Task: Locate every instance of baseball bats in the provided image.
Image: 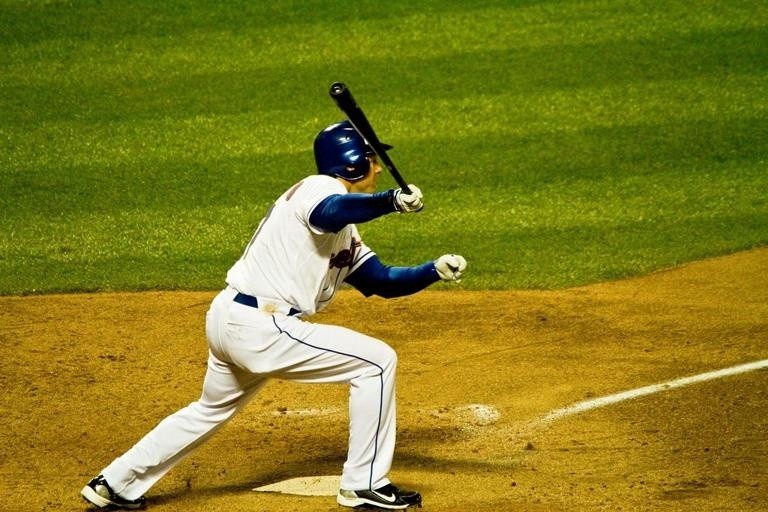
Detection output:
[329,81,412,195]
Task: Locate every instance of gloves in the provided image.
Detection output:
[393,183,425,214]
[434,253,468,285]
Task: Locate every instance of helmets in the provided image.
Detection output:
[313,120,394,183]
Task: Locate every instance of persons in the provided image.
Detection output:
[80,120,470,511]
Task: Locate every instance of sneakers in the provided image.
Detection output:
[336,482,423,511]
[80,471,146,509]
